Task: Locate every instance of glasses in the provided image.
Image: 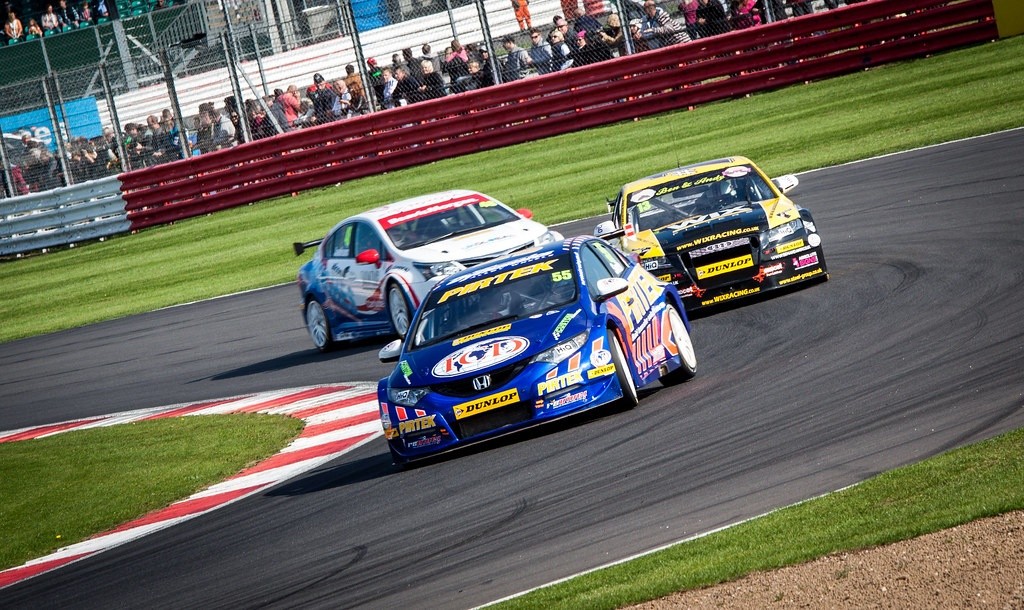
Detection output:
[553,36,557,38]
[556,24,566,28]
[531,34,540,38]
[576,38,581,41]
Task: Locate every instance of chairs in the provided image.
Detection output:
[8,0,172,45]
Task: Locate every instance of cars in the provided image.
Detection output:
[292,189,565,352]
[593,155,828,316]
[377,235,697,466]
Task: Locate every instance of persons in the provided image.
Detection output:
[190,45,447,177]
[706,181,737,209]
[440,0,626,131]
[0,109,192,199]
[478,291,511,317]
[5,0,110,45]
[629,0,926,89]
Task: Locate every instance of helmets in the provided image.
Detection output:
[478,285,521,323]
[386,224,408,247]
[706,181,738,211]
[629,19,643,28]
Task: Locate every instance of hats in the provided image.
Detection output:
[314,73,324,84]
[368,57,377,66]
[479,45,488,53]
[576,30,587,38]
